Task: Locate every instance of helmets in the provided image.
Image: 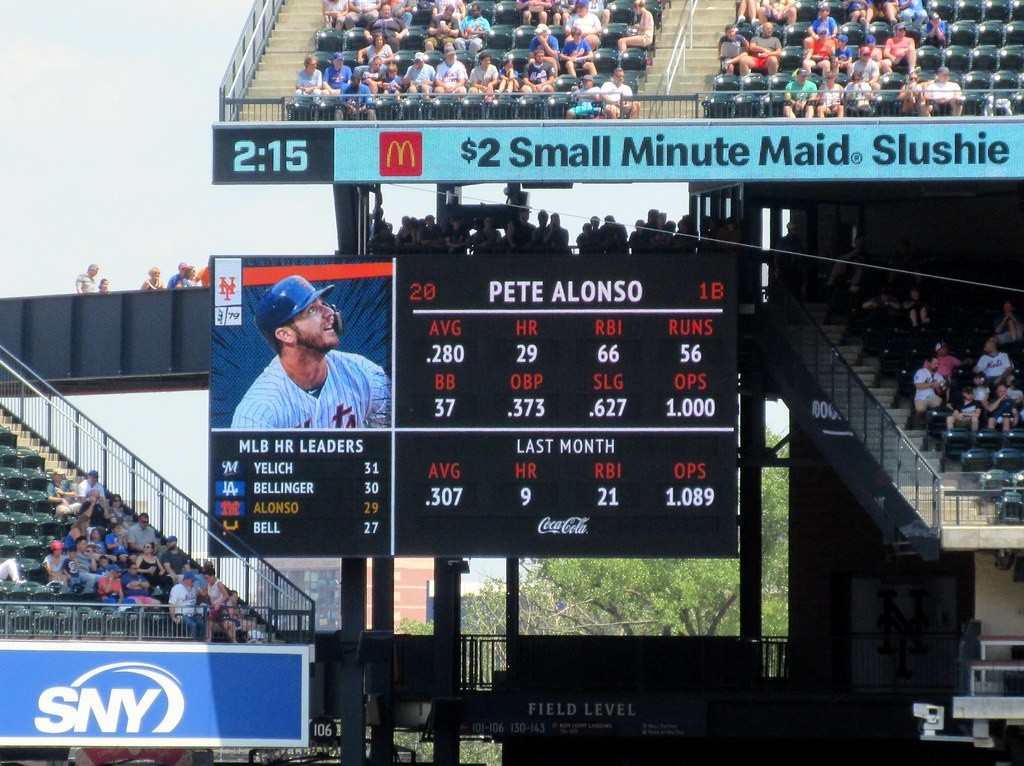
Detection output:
[251,273,335,334]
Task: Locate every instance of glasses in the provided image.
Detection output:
[864,53,870,56]
[910,78,918,82]
[899,28,906,31]
[143,545,150,549]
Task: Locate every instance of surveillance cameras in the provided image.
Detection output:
[913,704,939,718]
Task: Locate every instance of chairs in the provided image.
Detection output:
[285,0,665,129]
[813,301,1024,527]
[0,420,174,631]
[702,0,1024,117]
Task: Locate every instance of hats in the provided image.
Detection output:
[575,0,588,7]
[165,535,177,542]
[50,541,63,550]
[444,46,457,56]
[333,52,345,61]
[817,4,831,10]
[895,24,906,31]
[414,51,425,62]
[178,261,188,270]
[183,571,200,581]
[534,24,549,33]
[796,68,807,76]
[837,35,848,42]
[817,25,827,34]
[865,34,876,46]
[108,563,123,572]
[860,47,870,54]
[928,10,940,20]
[937,67,950,75]
[87,470,100,478]
[578,74,594,82]
[52,467,66,475]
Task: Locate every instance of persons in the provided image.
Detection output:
[75,264,99,293]
[0,558,27,584]
[567,75,607,119]
[516,0,611,34]
[600,67,641,119]
[167,263,198,288]
[783,0,965,117]
[287,46,558,106]
[43,468,262,644]
[576,210,744,254]
[718,21,782,76]
[322,0,418,53]
[736,0,797,33]
[368,206,572,255]
[564,0,602,52]
[194,266,210,286]
[98,278,110,293]
[559,27,597,75]
[618,0,654,52]
[528,24,560,74]
[228,274,391,429]
[141,267,165,289]
[334,73,376,120]
[772,224,1024,433]
[422,0,491,51]
